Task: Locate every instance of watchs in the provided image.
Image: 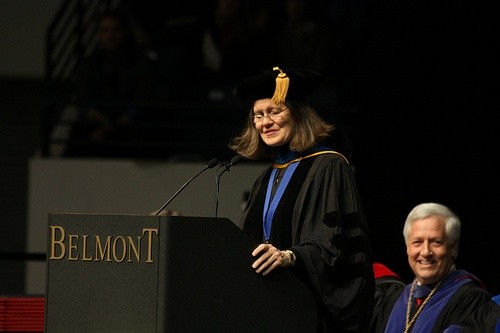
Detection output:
[286,249,297,265]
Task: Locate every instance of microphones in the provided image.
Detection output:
[156,159,217,215]
[215,155,242,218]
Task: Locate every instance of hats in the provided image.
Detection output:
[240,70,321,101]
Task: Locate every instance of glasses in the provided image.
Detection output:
[252,107,289,118]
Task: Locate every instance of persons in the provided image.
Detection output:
[73,0,332,154]
[364,202,500,332]
[228,71,377,333]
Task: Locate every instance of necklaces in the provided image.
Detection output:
[405,278,441,333]
